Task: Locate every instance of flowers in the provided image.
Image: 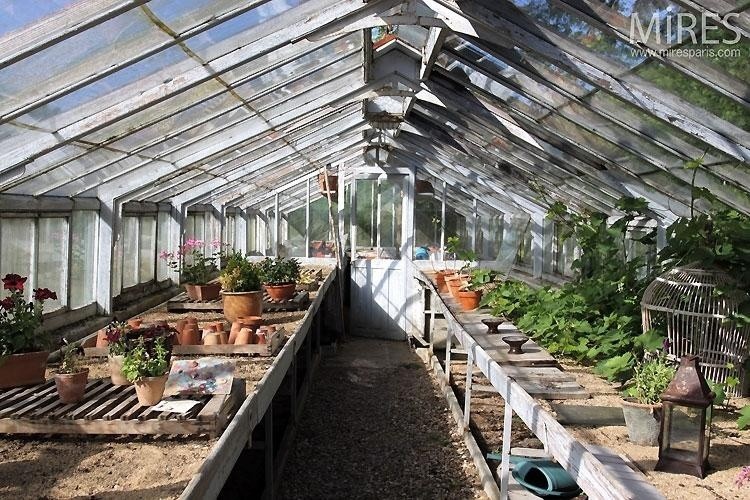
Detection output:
[618,337,681,405]
[159,238,223,285]
[0,273,57,354]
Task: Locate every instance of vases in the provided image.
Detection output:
[0,351,50,388]
[184,282,203,301]
[620,401,663,446]
[194,285,219,300]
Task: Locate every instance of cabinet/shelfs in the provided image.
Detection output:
[0,261,336,499]
[415,274,656,500]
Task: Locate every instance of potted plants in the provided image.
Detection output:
[444,247,482,299]
[52,337,90,403]
[458,266,506,311]
[258,255,304,300]
[103,321,134,385]
[437,231,463,293]
[216,247,263,325]
[118,334,173,407]
